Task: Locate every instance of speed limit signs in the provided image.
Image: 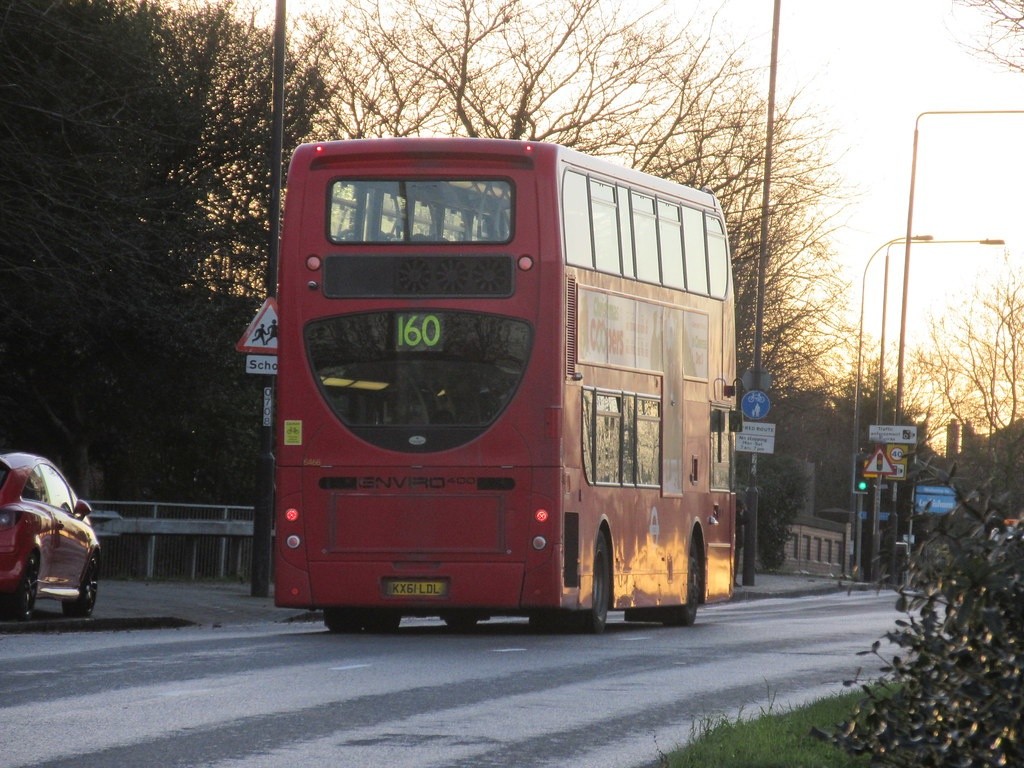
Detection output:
[884,444,908,481]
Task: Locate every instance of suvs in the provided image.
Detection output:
[0,451,103,622]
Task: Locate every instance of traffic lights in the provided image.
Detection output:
[852,453,869,495]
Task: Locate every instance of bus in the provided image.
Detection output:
[265,138,748,636]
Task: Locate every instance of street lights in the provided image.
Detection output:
[843,233,937,582]
[867,236,1007,581]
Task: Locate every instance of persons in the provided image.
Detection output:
[733,499,752,589]
